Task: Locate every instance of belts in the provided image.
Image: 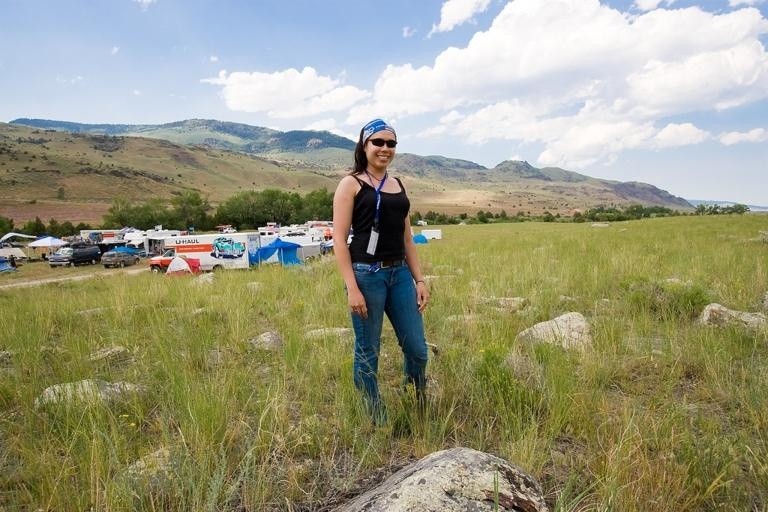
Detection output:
[375,258,406,271]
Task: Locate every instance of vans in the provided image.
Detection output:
[49,245,101,268]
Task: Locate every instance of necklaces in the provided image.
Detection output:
[367,170,388,182]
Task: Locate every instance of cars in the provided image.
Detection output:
[100,250,140,268]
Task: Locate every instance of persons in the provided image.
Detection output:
[332,120,430,437]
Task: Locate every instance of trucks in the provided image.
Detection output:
[149,232,262,280]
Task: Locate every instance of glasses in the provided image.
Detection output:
[367,137,398,148]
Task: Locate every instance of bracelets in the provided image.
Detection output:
[416,280,425,284]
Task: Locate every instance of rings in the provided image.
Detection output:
[355,310,358,312]
